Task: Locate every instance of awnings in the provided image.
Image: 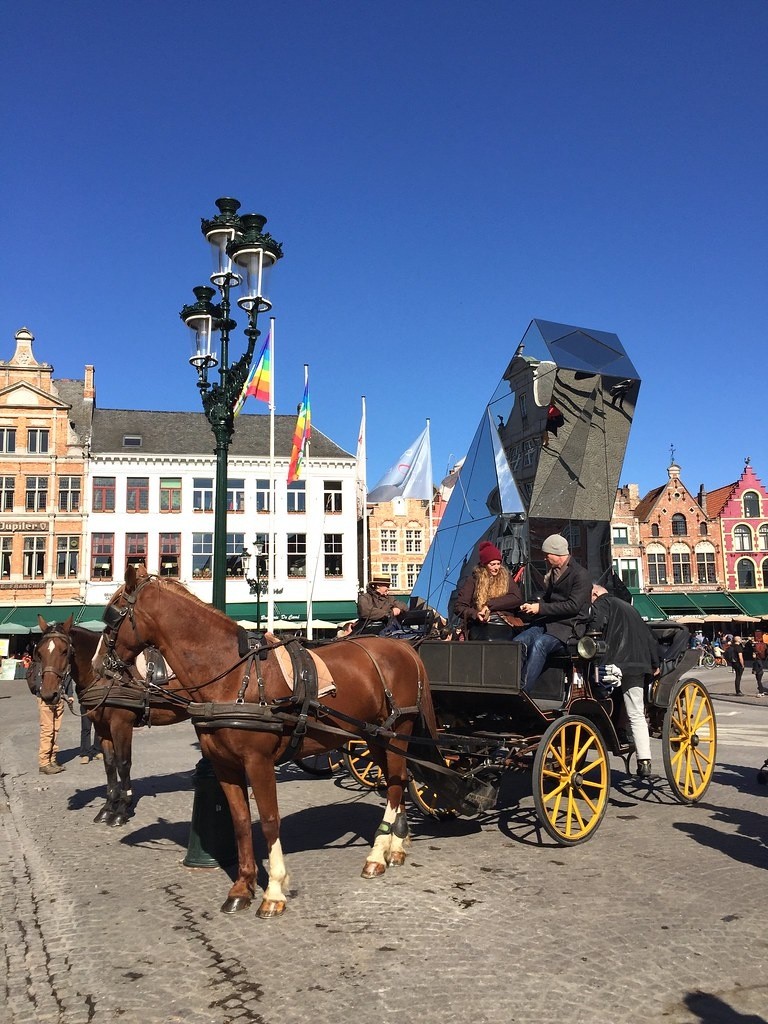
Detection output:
[629,593,768,623]
[0,606,107,634]
[226,603,360,629]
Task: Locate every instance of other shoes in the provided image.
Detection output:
[756,693,764,698]
[39,764,61,775]
[95,753,103,760]
[80,756,90,764]
[51,761,66,771]
[736,691,746,696]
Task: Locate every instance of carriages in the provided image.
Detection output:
[31,564,718,921]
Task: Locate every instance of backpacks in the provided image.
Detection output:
[723,646,735,666]
[26,661,38,694]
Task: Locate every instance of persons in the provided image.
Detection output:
[691,630,765,698]
[513,534,593,696]
[453,541,524,641]
[336,622,353,638]
[79,701,104,764]
[22,653,32,680]
[9,654,15,659]
[35,643,74,775]
[587,584,661,778]
[351,575,409,636]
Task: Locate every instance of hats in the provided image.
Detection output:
[541,533,570,555]
[714,642,719,645]
[478,541,502,566]
[369,574,392,585]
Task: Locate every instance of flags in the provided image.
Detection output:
[286,373,311,486]
[367,425,433,502]
[233,329,272,419]
[355,412,366,503]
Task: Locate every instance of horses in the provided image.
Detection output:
[35,610,256,827]
[91,563,440,919]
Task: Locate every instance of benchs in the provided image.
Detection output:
[645,620,691,676]
[520,637,580,700]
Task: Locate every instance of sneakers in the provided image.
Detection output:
[637,759,652,776]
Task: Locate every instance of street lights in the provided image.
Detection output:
[179,195,285,621]
